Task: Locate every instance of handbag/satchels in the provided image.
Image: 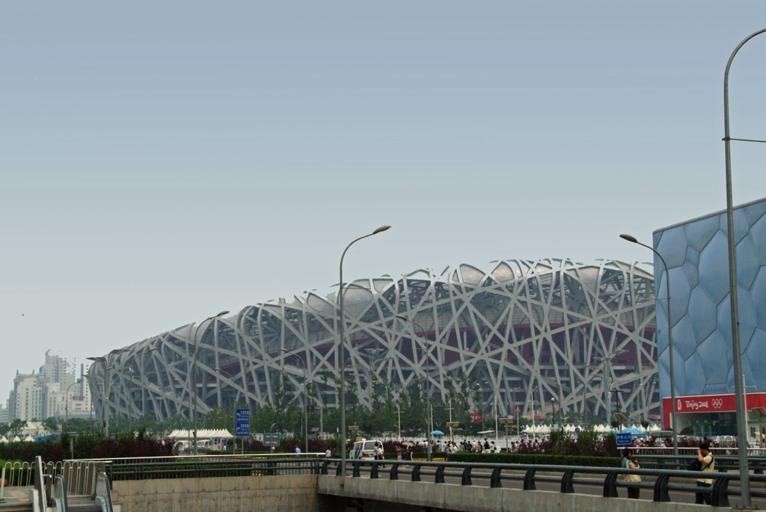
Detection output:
[624,460,642,483]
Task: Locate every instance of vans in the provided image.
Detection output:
[349,439,386,466]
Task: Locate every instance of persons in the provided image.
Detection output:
[374,442,385,468]
[621,448,641,499]
[325,445,332,458]
[628,436,766,454]
[393,437,545,461]
[695,441,714,504]
[295,444,302,467]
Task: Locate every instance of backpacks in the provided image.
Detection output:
[689,454,714,471]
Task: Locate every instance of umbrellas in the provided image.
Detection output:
[431,430,445,439]
[753,432,766,440]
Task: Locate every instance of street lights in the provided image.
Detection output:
[278,346,308,458]
[394,314,432,465]
[618,230,682,470]
[126,346,161,439]
[213,365,245,453]
[63,378,81,421]
[336,223,393,469]
[549,396,556,426]
[190,309,231,456]
